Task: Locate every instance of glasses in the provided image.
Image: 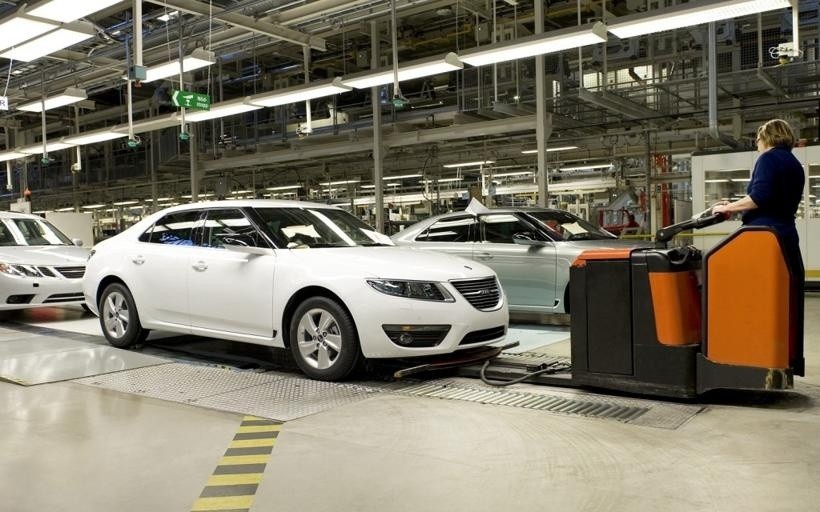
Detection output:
[755,139,760,145]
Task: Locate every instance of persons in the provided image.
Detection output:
[711,119,804,388]
[627,214,641,227]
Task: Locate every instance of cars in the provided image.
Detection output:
[82,199,509,381]
[1,210,94,316]
[391,206,652,315]
